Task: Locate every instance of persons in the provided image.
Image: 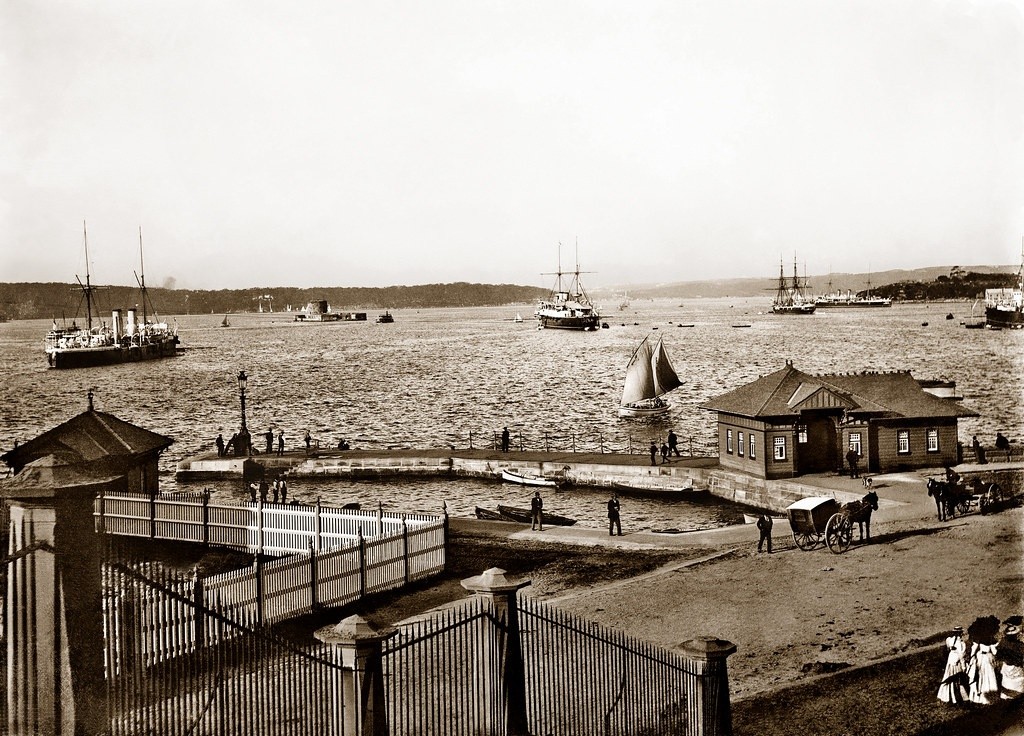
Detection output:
[650,430,680,465]
[757,508,774,554]
[846,450,858,479]
[277,432,285,456]
[266,428,273,453]
[532,492,544,531]
[972,436,980,451]
[936,626,1024,705]
[607,493,624,536]
[304,430,311,448]
[995,432,1009,449]
[249,478,287,504]
[502,427,509,452]
[216,434,224,457]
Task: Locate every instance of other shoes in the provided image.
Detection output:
[539,529,545,531]
[667,455,671,457]
[610,534,616,536]
[768,550,773,554]
[652,463,656,466]
[677,454,680,457]
[758,547,761,549]
[532,528,534,530]
[618,534,625,536]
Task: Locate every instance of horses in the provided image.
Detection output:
[927,477,1005,522]
[837,492,878,545]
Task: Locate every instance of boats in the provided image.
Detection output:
[290,495,361,514]
[501,464,572,488]
[474,505,519,523]
[813,262,894,308]
[497,502,578,527]
[375,310,394,324]
[983,234,1024,330]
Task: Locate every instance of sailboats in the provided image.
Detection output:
[532,233,601,331]
[760,249,818,316]
[42,215,181,371]
[616,330,689,420]
[511,312,525,324]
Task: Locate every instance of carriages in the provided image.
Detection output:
[927,474,1004,522]
[786,491,879,555]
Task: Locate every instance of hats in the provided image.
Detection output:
[503,427,507,429]
[535,492,540,495]
[953,626,963,632]
[1005,626,1020,635]
[651,442,655,444]
[669,430,673,432]
